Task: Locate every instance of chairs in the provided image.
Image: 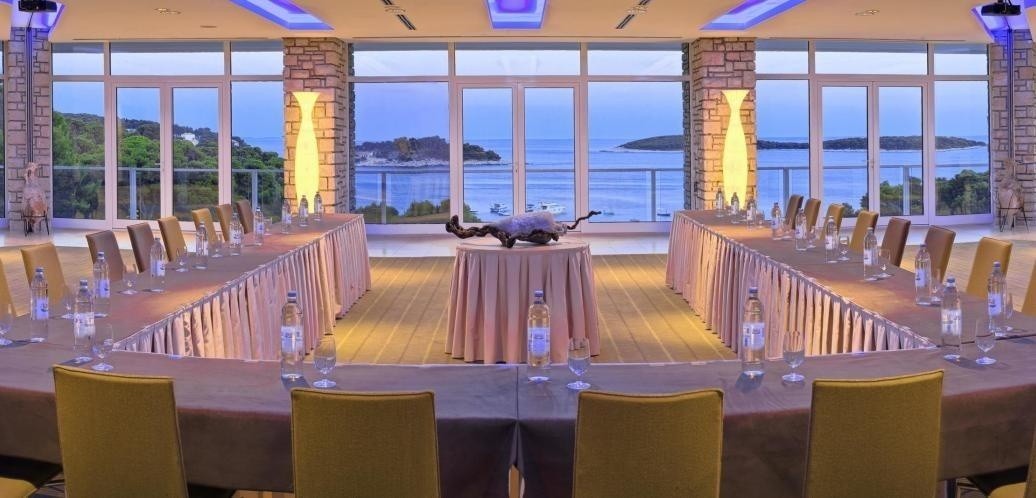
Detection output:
[571,389,724,497]
[51,364,189,498]
[989,428,1036,496]
[804,368,943,498]
[783,194,1036,317]
[0,477,38,498]
[0,199,255,318]
[289,386,440,498]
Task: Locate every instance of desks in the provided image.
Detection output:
[0,213,372,361]
[1,348,519,497]
[665,210,1036,358]
[514,335,1036,497]
[442,241,602,364]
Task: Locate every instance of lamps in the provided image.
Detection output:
[719,88,752,211]
[292,90,322,216]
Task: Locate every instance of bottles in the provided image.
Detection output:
[0,192,337,390]
[771,202,783,241]
[741,287,766,377]
[731,191,740,224]
[796,207,809,250]
[717,190,725,217]
[864,227,878,281]
[528,291,550,383]
[914,244,933,305]
[940,278,963,359]
[987,262,1007,337]
[825,215,839,265]
[748,198,756,230]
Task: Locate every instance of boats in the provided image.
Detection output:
[656,211,670,216]
[491,201,566,216]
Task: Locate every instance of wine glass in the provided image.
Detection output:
[782,332,806,382]
[1006,293,1013,331]
[838,236,850,261]
[806,225,816,248]
[878,248,892,280]
[931,269,942,303]
[726,203,791,233]
[566,337,591,390]
[973,318,996,366]
